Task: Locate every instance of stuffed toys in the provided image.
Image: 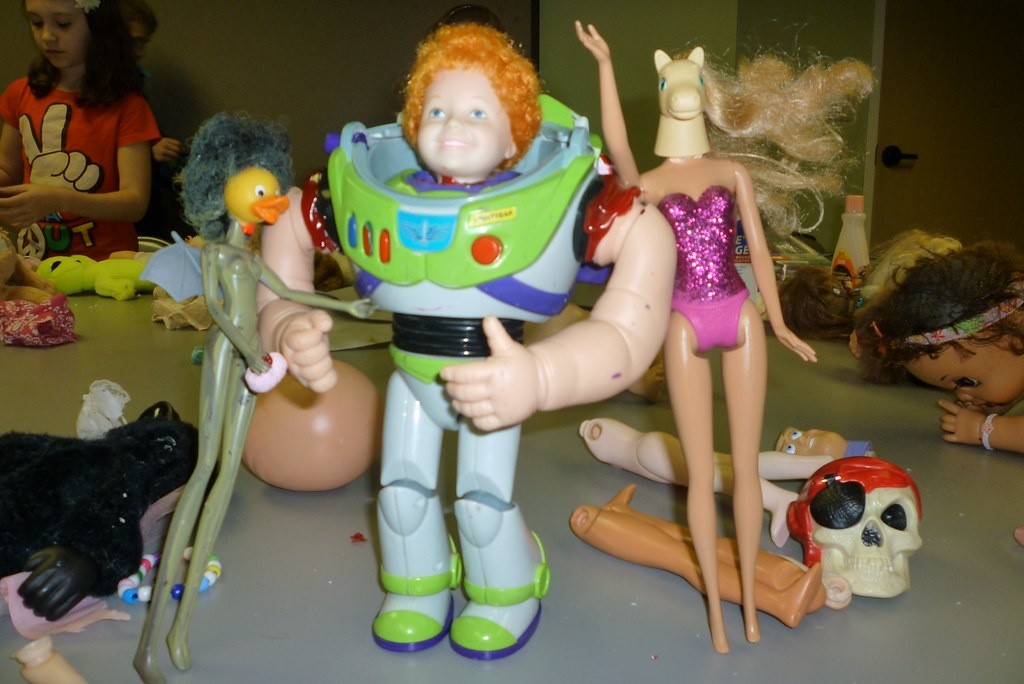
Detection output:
[35,254,157,301]
[0,400,219,621]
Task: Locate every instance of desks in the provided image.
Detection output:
[0,289,1024,683]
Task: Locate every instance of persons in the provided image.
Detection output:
[153,19,1024,658]
[0,0,159,265]
[110,1,198,247]
